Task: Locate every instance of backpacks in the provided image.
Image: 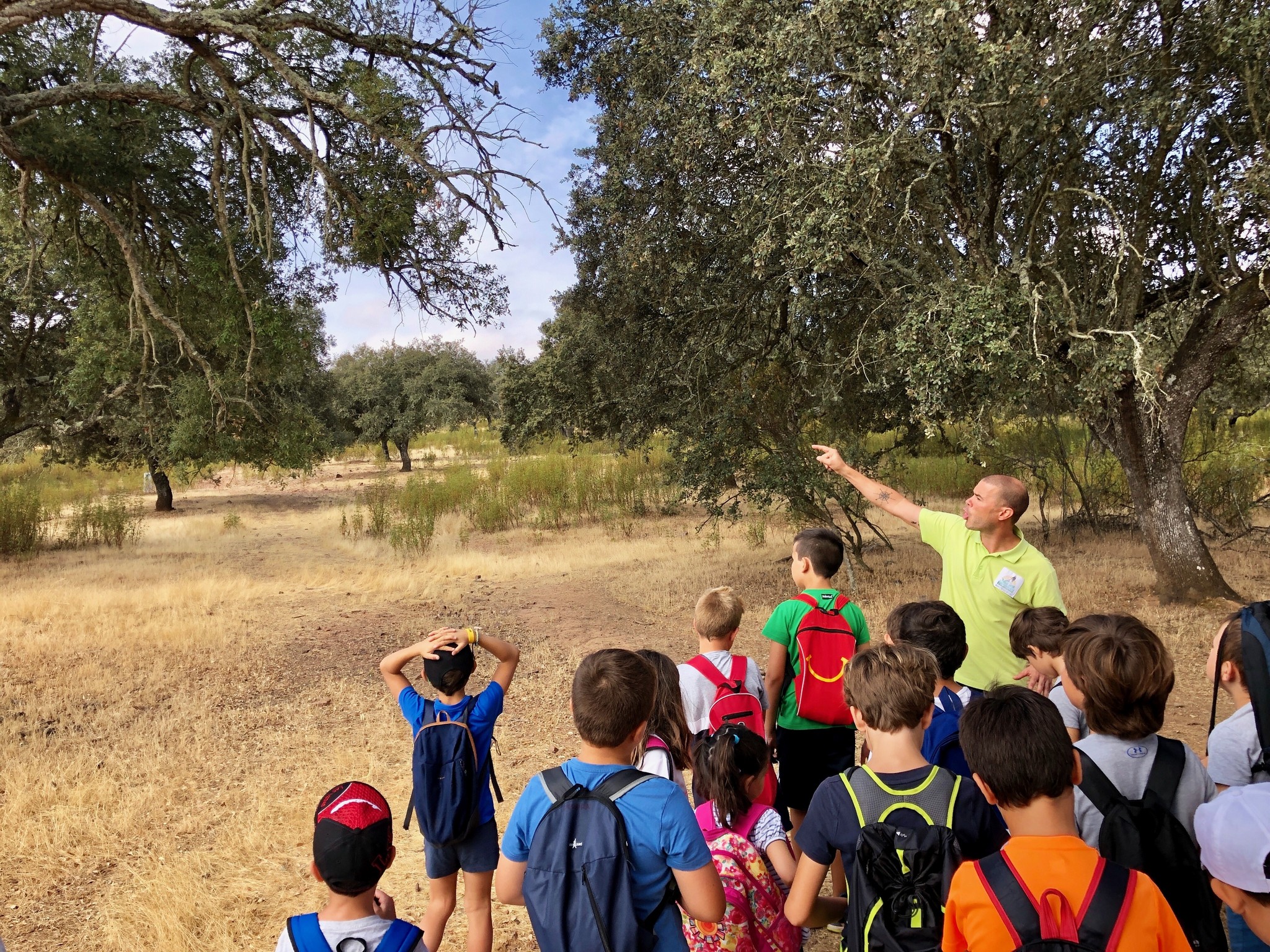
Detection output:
[974,850,1137,952]
[674,799,802,952]
[790,594,856,724]
[522,767,674,952]
[1073,735,1228,951]
[685,655,766,739]
[920,685,973,777]
[839,764,964,952]
[412,695,479,846]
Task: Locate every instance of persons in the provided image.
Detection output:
[276,780,431,952]
[380,627,519,952]
[496,528,1270,952]
[813,446,1067,697]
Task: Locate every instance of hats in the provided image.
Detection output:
[1193,781,1270,892]
[313,781,393,893]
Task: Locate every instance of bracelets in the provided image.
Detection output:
[465,628,474,645]
[472,627,483,653]
